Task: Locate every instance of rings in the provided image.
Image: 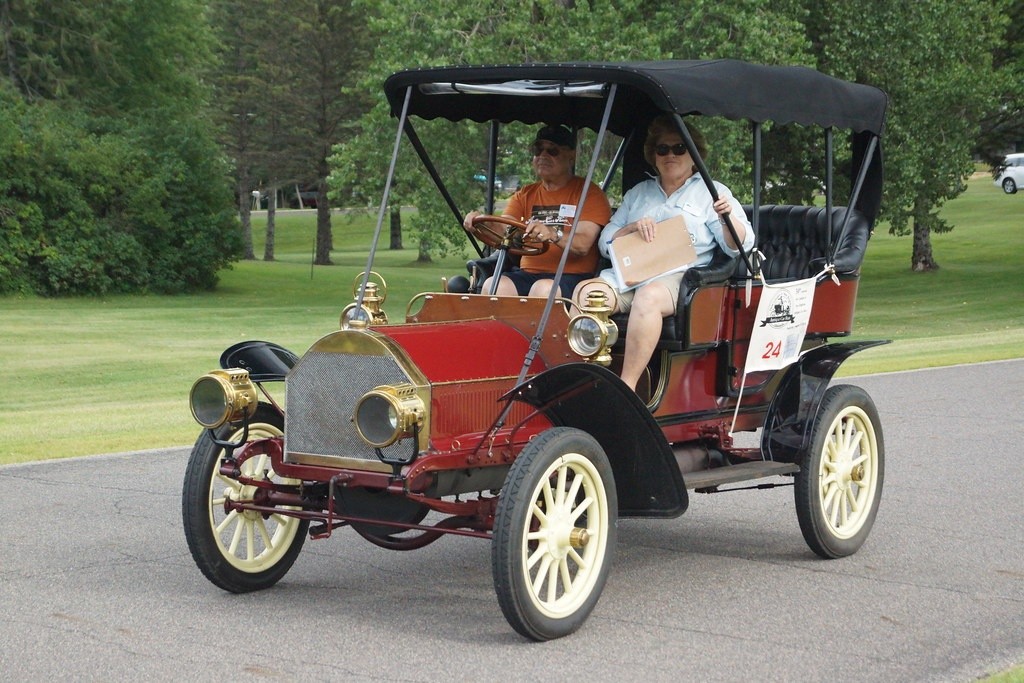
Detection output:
[539,233,544,237]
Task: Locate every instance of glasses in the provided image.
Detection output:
[533,146,569,157]
[654,144,687,156]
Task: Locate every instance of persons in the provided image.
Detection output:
[567,115,755,394]
[463,125,612,312]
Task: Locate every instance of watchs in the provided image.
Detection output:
[719,218,727,225]
[552,226,564,244]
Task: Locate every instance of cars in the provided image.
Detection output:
[993,153,1024,195]
[180,58,894,646]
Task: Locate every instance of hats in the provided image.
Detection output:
[528,125,576,150]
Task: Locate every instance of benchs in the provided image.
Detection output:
[743,203,869,338]
[465,223,739,346]
